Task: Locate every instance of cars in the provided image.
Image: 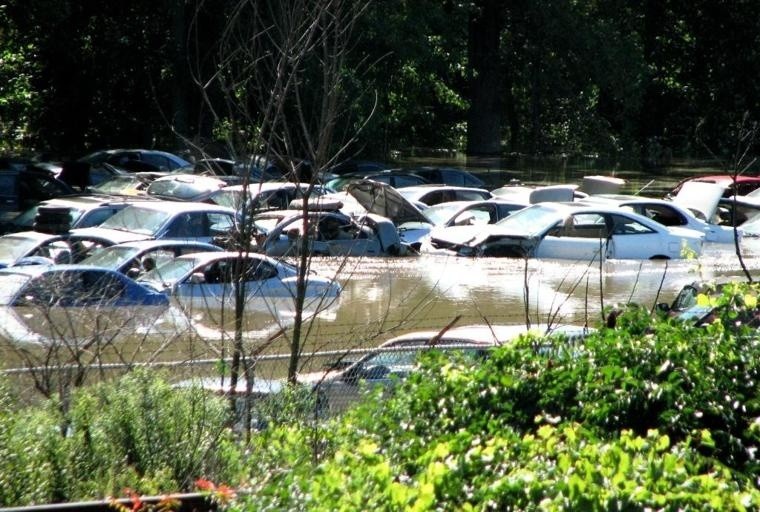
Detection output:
[647,278,759,334]
[314,324,598,415]
